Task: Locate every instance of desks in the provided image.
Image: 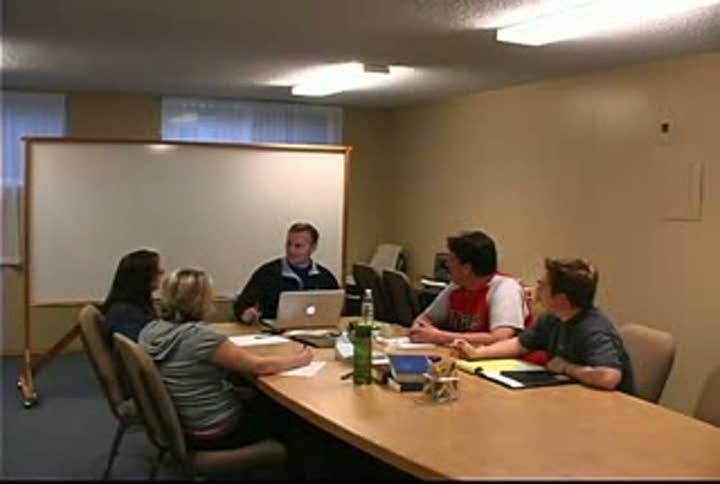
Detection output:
[207,314,718,481]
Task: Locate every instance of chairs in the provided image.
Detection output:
[353,260,380,293]
[381,266,412,323]
[112,331,289,481]
[693,366,719,430]
[76,303,141,481]
[616,321,682,405]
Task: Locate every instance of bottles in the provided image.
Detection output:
[361,289,374,328]
[355,328,372,389]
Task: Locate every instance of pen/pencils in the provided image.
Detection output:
[341,371,354,380]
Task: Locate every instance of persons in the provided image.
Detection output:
[136,265,310,449]
[94,249,169,420]
[448,258,634,396]
[411,230,548,366]
[234,223,341,323]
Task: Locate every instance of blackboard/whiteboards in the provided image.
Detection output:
[20,135,353,306]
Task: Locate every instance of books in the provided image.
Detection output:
[387,378,425,392]
[371,365,389,384]
[389,355,433,382]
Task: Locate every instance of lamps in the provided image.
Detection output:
[285,60,423,99]
[494,2,720,47]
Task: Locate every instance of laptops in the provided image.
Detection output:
[261,289,345,331]
